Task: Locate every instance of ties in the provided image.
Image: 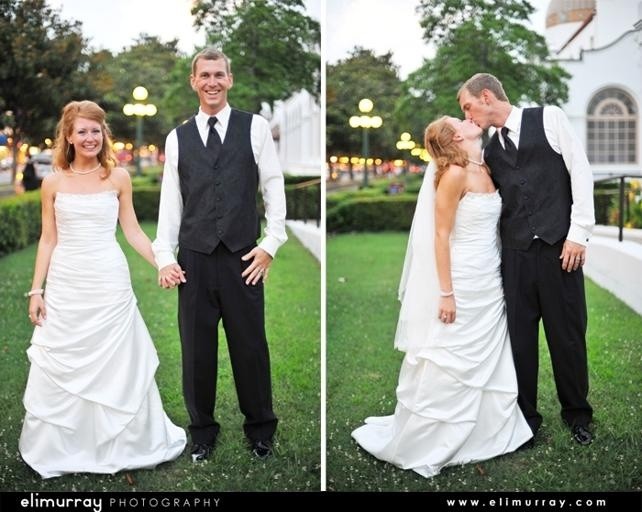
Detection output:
[501,126,517,165]
[205,117,223,159]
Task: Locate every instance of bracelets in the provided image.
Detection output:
[437,289,454,297]
[25,288,45,297]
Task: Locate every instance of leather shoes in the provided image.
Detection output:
[251,439,274,457]
[571,424,595,447]
[188,440,213,464]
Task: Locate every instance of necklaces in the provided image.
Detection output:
[467,160,484,166]
[70,163,101,174]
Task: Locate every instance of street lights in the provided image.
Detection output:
[124,85,157,178]
[349,98,382,190]
[396,131,415,175]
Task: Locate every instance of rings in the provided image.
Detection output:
[259,271,263,275]
[28,314,32,318]
[442,315,447,319]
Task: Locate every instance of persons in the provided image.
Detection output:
[22,155,38,192]
[351,114,536,477]
[16,99,188,480]
[457,72,597,445]
[151,46,288,462]
